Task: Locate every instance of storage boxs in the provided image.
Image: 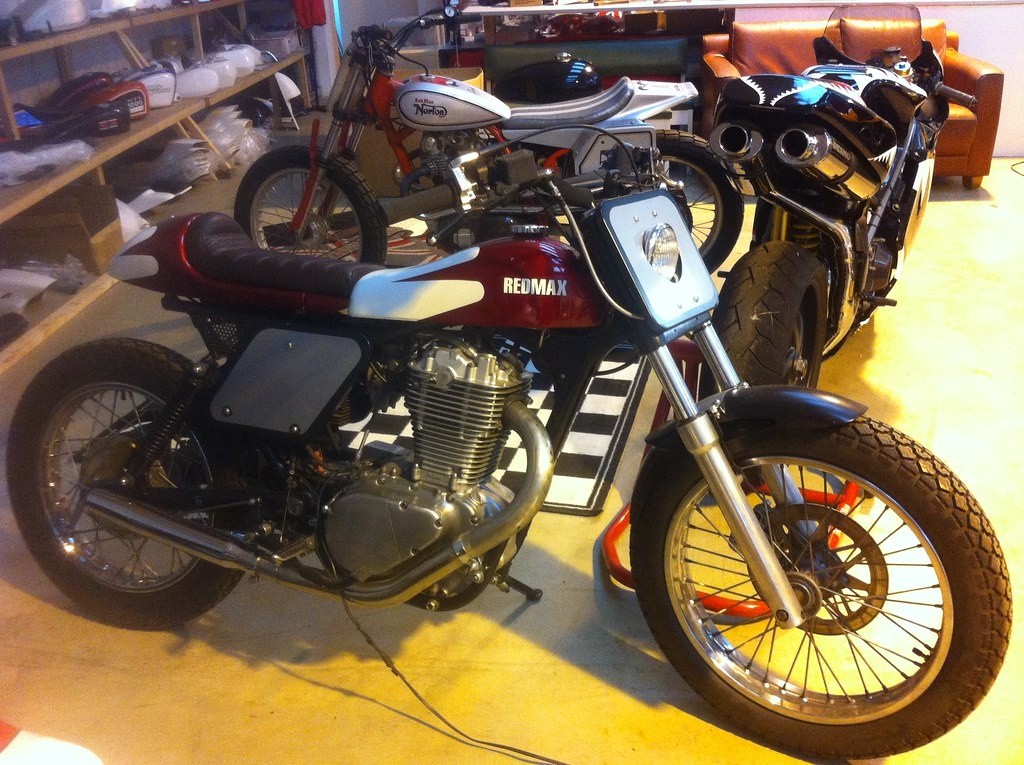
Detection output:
[244,24,303,58]
[2,183,125,276]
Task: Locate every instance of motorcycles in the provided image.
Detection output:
[234,0,746,276]
[6,124,1014,759]
[698,36,978,401]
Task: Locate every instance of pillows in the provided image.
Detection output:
[729,23,950,78]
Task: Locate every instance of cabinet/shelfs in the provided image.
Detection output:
[0,0,308,374]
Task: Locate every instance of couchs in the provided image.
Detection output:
[697,19,1005,188]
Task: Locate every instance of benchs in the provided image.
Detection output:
[485,35,688,109]
